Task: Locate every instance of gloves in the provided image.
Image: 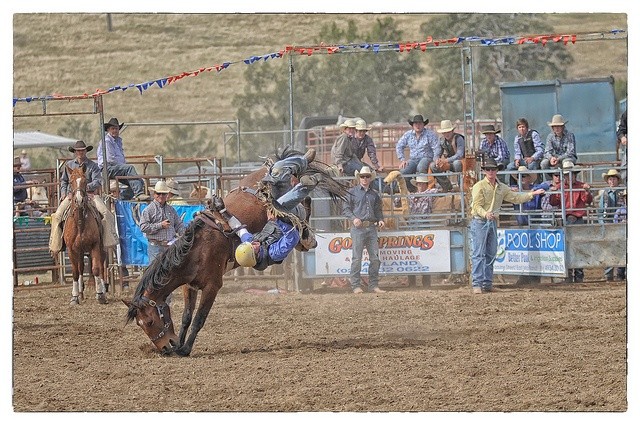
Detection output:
[213,194,233,222]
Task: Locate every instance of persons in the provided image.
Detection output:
[480,125,509,177]
[331,118,395,194]
[20,149,30,173]
[343,165,386,294]
[541,172,562,225]
[14,156,29,217]
[598,168,625,281]
[550,160,594,283]
[165,177,189,206]
[470,166,544,294]
[51,140,120,251]
[396,114,447,192]
[613,191,629,223]
[430,120,465,193]
[212,149,322,271]
[139,181,186,307]
[97,117,150,201]
[616,109,627,186]
[394,173,445,287]
[508,119,545,186]
[540,115,577,180]
[509,166,550,286]
[354,118,385,169]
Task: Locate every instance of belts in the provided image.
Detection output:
[362,221,374,227]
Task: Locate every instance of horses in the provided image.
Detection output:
[122,147,355,358]
[60,162,113,305]
[24,179,50,212]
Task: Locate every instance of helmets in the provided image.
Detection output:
[235,242,256,267]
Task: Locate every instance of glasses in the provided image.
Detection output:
[517,176,525,178]
[360,176,370,178]
[156,193,167,195]
[486,168,496,171]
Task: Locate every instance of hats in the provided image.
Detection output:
[356,120,370,130]
[14,157,24,166]
[547,115,568,126]
[410,174,433,187]
[602,169,621,184]
[103,118,124,132]
[437,120,456,133]
[408,115,429,126]
[355,166,376,181]
[340,119,357,127]
[482,125,501,134]
[511,166,537,183]
[481,160,503,171]
[166,179,179,195]
[558,161,581,174]
[548,173,560,177]
[69,141,93,153]
[148,181,170,193]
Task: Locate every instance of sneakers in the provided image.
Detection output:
[473,287,482,293]
[304,149,316,164]
[484,287,499,292]
[135,194,150,200]
[353,288,364,293]
[301,173,322,185]
[368,288,386,293]
[441,186,453,193]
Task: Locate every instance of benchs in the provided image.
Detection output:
[95,167,293,282]
[474,160,616,270]
[307,155,477,283]
